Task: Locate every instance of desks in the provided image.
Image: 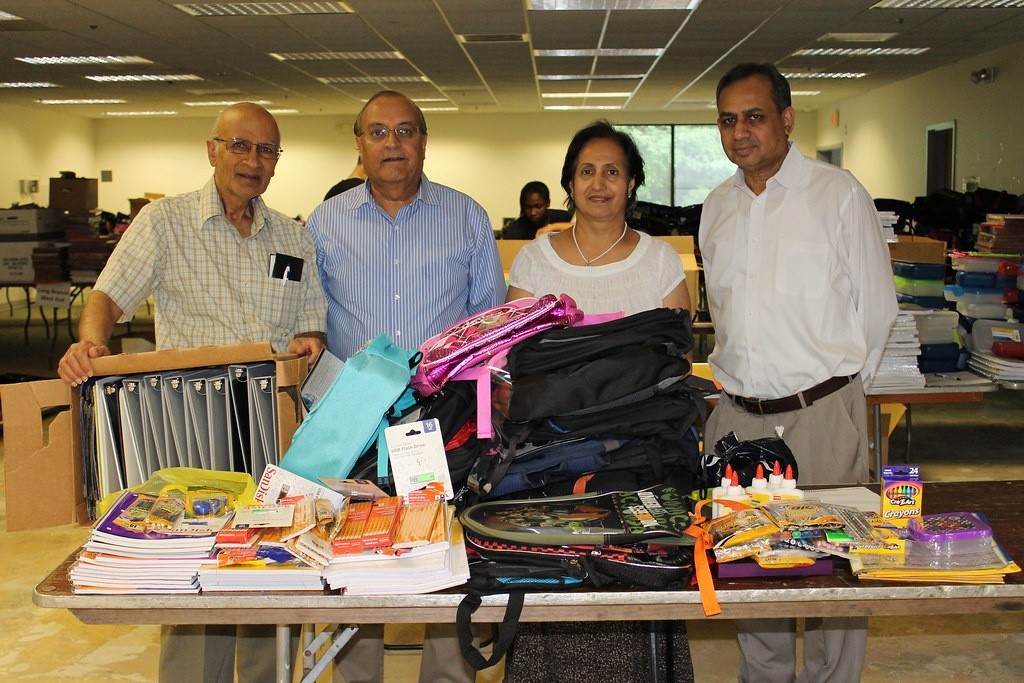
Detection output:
[32,477,1024,683]
[865,389,984,487]
[0,277,150,372]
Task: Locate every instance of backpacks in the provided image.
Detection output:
[276,294,704,589]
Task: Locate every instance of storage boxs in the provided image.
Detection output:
[0,339,310,533]
[128,199,150,223]
[888,233,947,266]
[49,177,98,210]
[0,241,70,284]
[0,209,60,234]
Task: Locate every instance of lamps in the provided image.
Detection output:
[971,66,994,84]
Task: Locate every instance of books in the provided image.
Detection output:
[878,212,900,244]
[66,490,471,596]
[867,302,933,395]
[966,350,1024,381]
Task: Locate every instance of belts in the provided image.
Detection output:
[721,372,859,415]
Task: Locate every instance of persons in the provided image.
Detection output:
[499,182,572,241]
[304,90,507,683]
[58,103,327,683]
[504,118,695,683]
[698,62,900,682]
[322,155,368,203]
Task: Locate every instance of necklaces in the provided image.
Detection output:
[572,221,628,267]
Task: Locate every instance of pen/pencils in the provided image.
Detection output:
[282,263,290,287]
[935,373,961,381]
[767,507,889,552]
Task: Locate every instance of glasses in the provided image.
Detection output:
[359,125,426,140]
[213,137,283,159]
